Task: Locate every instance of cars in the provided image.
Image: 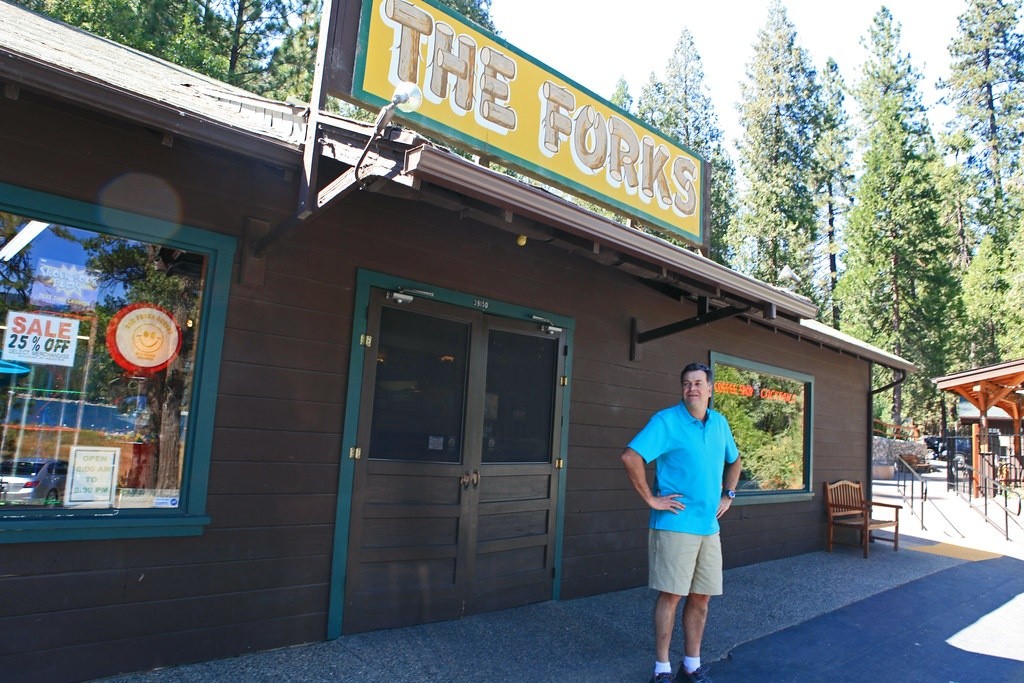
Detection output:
[919,435,963,461]
[0,459,68,507]
[940,437,1012,470]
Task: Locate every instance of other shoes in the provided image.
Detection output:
[650,672,674,683]
[675,661,713,683]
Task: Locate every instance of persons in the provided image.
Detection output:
[621,363,741,683]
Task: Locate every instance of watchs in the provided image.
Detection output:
[722,489,735,500]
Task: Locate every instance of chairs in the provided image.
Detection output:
[822,479,903,559]
[900,454,932,473]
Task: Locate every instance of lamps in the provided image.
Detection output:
[778,265,818,304]
[354,80,423,192]
